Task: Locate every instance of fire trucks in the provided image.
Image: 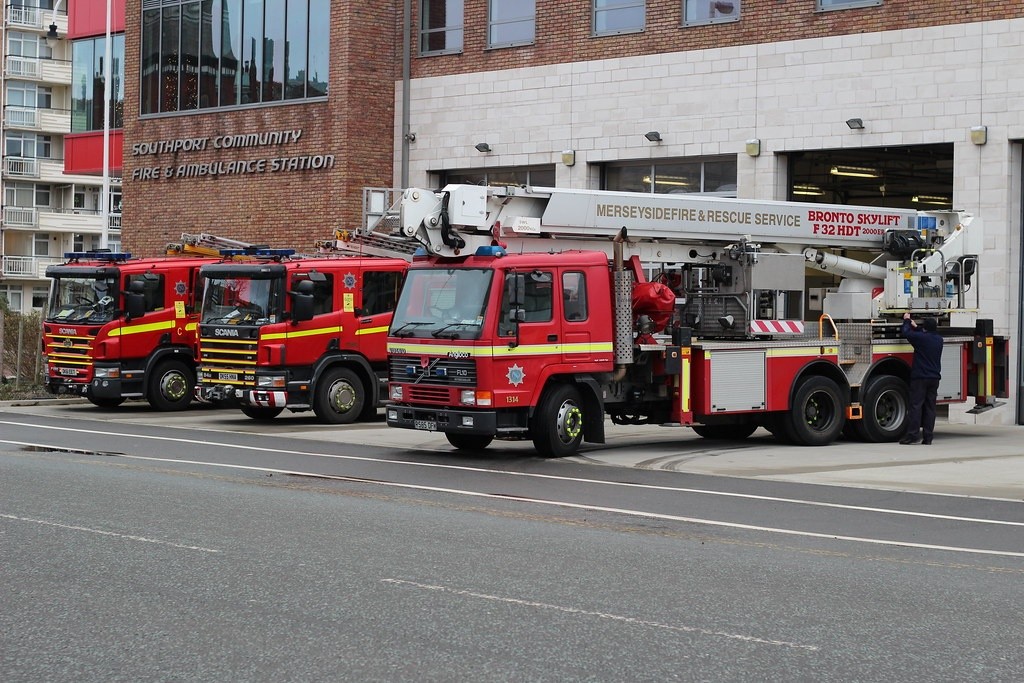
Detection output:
[384,184,1007,459]
[37,225,455,426]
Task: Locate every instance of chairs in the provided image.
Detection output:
[500,274,551,323]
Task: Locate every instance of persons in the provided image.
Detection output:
[901,313,944,446]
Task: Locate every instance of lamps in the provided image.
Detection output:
[969,126,988,147]
[744,138,761,157]
[474,142,493,153]
[845,117,865,131]
[560,149,576,167]
[644,130,663,143]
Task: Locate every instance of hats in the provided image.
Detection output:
[923,317,937,331]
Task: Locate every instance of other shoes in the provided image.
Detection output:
[900,433,920,444]
[922,438,932,445]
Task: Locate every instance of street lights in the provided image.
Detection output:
[43,0,112,250]
